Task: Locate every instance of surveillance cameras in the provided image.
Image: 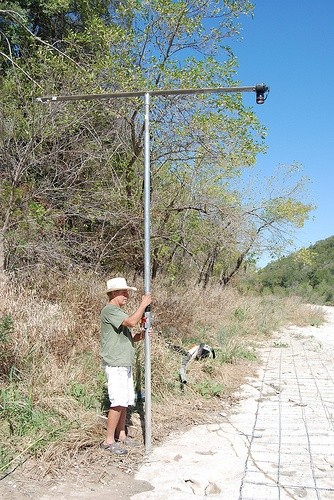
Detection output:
[256,91,264,103]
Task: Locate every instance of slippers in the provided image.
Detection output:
[117,437,140,449]
[100,442,128,455]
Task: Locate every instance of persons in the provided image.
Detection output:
[99,278,153,454]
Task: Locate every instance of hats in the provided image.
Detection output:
[103,277,137,294]
